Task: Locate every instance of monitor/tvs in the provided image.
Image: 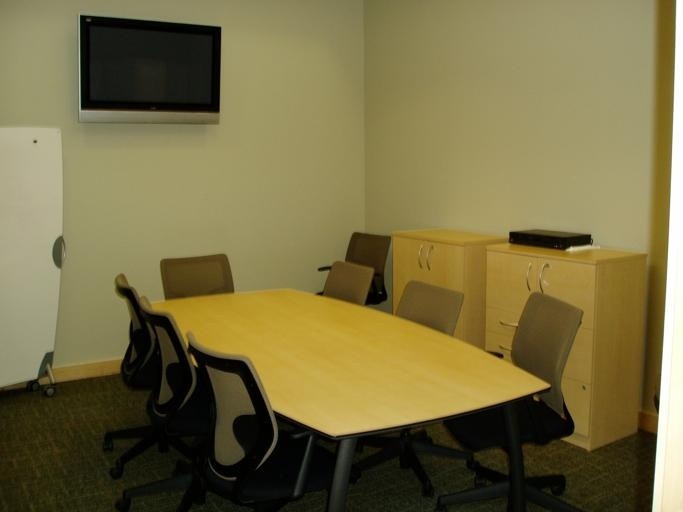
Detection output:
[77,13,223,125]
